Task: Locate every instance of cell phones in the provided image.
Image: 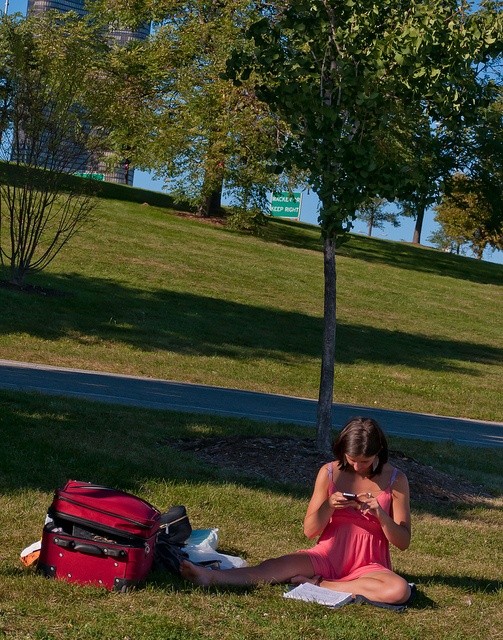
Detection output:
[343,493,356,500]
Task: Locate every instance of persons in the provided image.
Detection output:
[178,415,411,606]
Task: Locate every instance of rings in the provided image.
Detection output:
[368,493,372,497]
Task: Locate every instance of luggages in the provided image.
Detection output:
[34,478,161,594]
